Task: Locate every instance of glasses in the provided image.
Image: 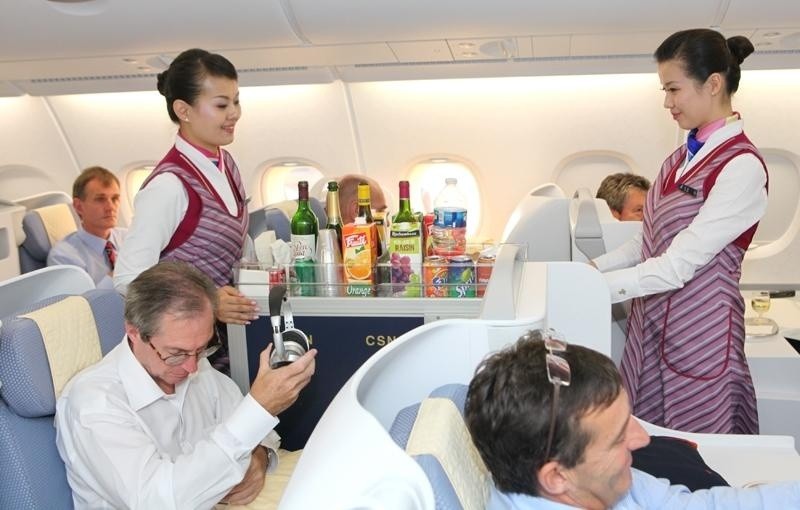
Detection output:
[544,328,571,462]
[146,324,223,365]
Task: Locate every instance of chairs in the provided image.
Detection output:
[389,384,493,510]
[248,197,327,242]
[0,286,127,509]
[19,203,78,274]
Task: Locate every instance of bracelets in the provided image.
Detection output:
[263,446,273,468]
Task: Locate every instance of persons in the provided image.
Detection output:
[47,166,126,292]
[587,28,768,434]
[463,326,800,509]
[336,176,386,224]
[55,259,317,510]
[596,173,652,222]
[106,49,258,376]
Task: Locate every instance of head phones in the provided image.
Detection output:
[265,282,309,366]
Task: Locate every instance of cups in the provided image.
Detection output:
[316,228,342,296]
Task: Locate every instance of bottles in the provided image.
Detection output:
[290,181,318,262]
[356,182,379,258]
[394,179,417,223]
[324,182,344,256]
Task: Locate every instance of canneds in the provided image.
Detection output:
[423,255,450,298]
[477,256,495,298]
[267,267,285,292]
[450,255,476,298]
[290,257,316,296]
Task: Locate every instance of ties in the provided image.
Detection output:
[105,242,116,269]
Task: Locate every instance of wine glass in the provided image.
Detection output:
[752,291,770,325]
